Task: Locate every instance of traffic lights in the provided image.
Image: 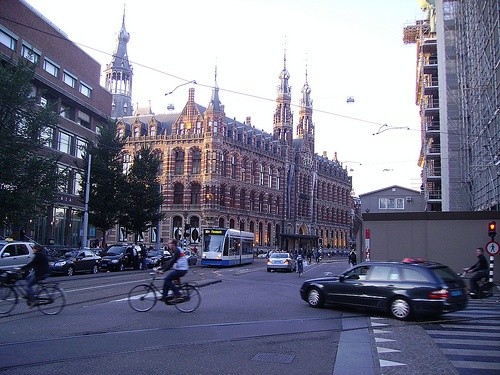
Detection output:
[489,221,497,237]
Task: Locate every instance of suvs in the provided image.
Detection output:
[0,238,49,280]
[184,250,197,266]
[146,250,172,269]
[298,257,466,321]
[97,244,140,271]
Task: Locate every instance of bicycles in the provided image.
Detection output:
[127,266,201,313]
[0,265,66,315]
[457,267,494,299]
[297,261,303,277]
[306,257,311,265]
[315,256,320,263]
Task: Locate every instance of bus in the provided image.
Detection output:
[201,228,254,267]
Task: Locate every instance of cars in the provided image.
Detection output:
[47,247,101,276]
[266,252,296,273]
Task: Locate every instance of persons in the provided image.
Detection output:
[347,249,358,274]
[15,244,49,309]
[138,243,148,269]
[266,247,343,273]
[465,248,490,296]
[157,239,189,302]
